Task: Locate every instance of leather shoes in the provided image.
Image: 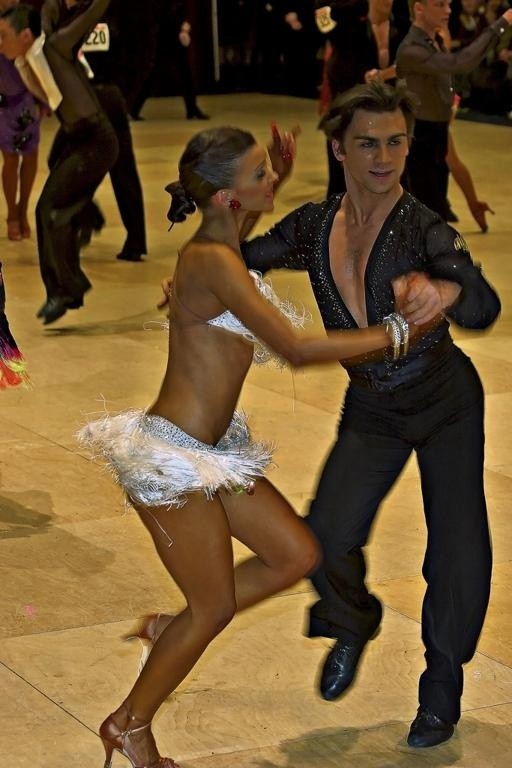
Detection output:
[34,291,85,326]
[184,106,211,121]
[313,623,383,702]
[406,701,458,747]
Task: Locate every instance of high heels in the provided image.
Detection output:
[475,200,497,233]
[97,711,182,767]
[117,611,178,704]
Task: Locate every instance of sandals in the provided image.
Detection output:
[4,210,32,241]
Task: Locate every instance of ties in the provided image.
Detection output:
[19,56,55,116]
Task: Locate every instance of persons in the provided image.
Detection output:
[1,0,212,392]
[264,0,512,234]
[156,79,503,749]
[76,115,450,765]
